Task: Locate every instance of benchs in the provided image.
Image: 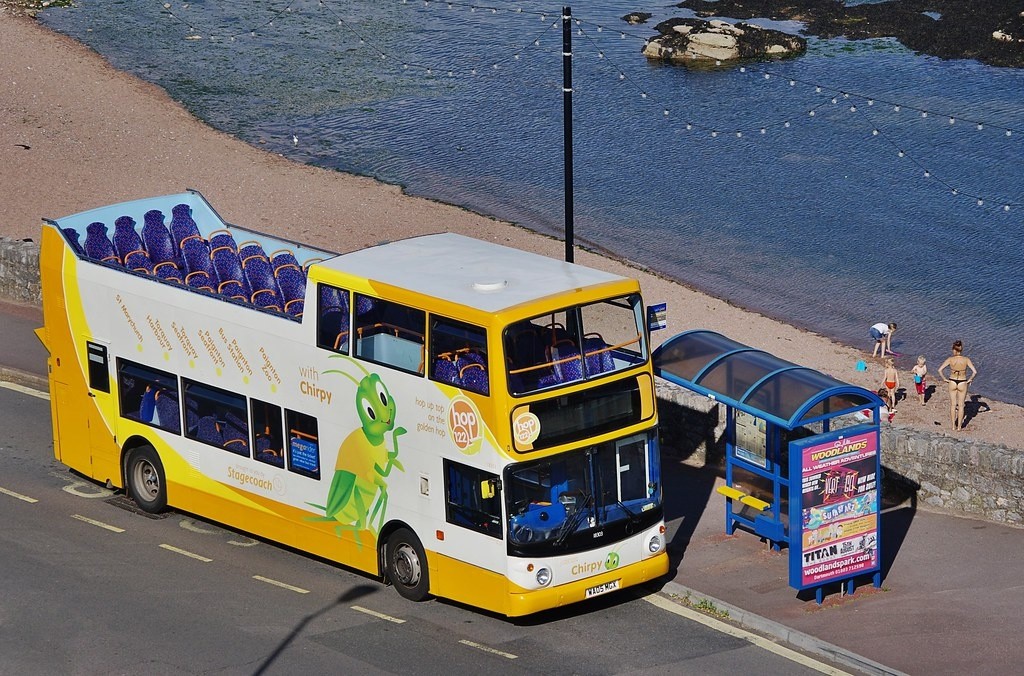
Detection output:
[715,485,774,514]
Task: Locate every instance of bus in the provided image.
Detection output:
[33,188,669,619]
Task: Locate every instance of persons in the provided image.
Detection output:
[938,341,978,432]
[870,321,927,425]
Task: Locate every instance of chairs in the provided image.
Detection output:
[242,255,279,295]
[141,210,174,263]
[83,222,117,262]
[218,279,250,302]
[208,230,238,255]
[112,216,145,266]
[180,235,213,275]
[237,241,270,268]
[169,204,201,256]
[250,257,378,346]
[100,257,122,267]
[431,323,616,506]
[269,249,303,280]
[124,249,153,273]
[138,380,285,466]
[210,247,246,282]
[62,227,88,261]
[186,270,216,291]
[154,263,183,283]
